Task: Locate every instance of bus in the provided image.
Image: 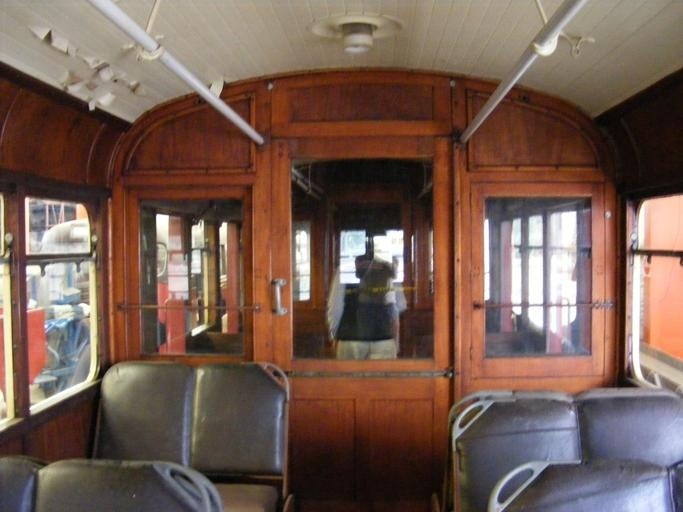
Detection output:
[0,192,227,417]
[623,194,682,402]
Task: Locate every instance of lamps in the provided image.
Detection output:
[312,13,404,57]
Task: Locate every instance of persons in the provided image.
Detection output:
[326,261,407,360]
[355,228,400,355]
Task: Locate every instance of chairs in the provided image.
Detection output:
[0,361,294,512]
[422,384,681,512]
